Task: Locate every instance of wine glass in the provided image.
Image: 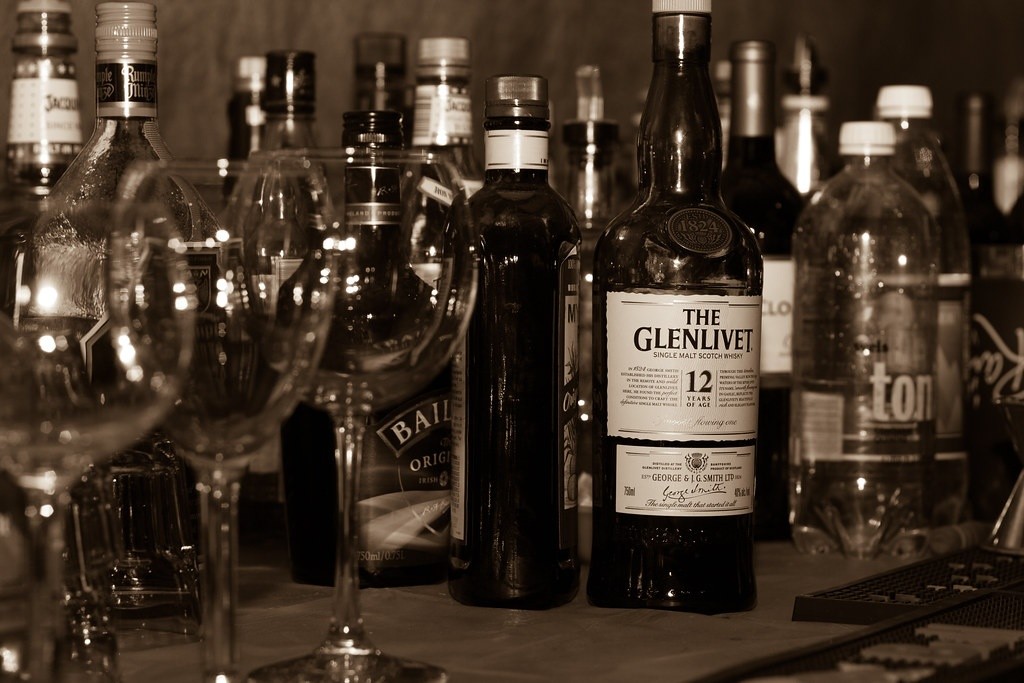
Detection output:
[1,135,481,683]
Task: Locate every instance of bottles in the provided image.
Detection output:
[0,0,93,335]
[219,0,1024,616]
[37,2,234,430]
